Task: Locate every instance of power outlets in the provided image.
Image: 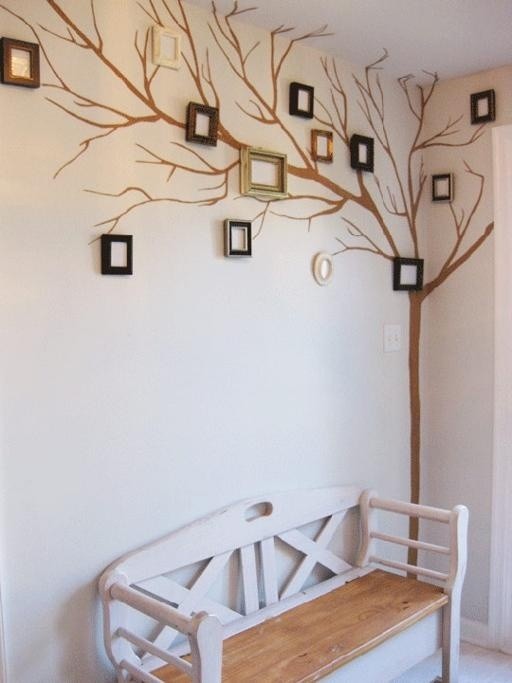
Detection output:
[383,324,402,353]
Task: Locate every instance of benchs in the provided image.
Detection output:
[100,484,470,683]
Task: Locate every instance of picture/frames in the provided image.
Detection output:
[430,173,454,203]
[100,234,132,275]
[313,252,333,286]
[0,36,40,88]
[224,219,253,258]
[350,133,374,173]
[288,81,314,118]
[392,256,424,291]
[311,129,333,164]
[186,101,219,147]
[239,145,289,198]
[151,26,181,70]
[470,88,496,124]
